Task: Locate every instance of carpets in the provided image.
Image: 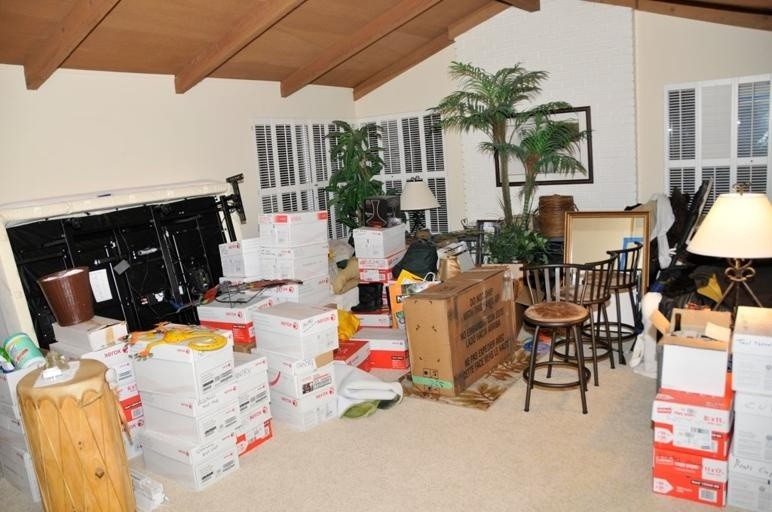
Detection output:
[398,335,550,412]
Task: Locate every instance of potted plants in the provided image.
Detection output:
[326,120,398,311]
[427,60,594,355]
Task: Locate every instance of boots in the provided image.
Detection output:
[369,282,384,307]
[351,283,376,311]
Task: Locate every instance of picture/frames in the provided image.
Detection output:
[492,106,594,187]
[562,209,651,330]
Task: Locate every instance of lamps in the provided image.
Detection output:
[400,178,437,236]
[686,191,771,314]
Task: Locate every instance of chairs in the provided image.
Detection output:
[519,264,594,415]
[582,239,644,343]
[552,254,623,387]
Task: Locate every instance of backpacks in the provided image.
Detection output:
[391,242,438,280]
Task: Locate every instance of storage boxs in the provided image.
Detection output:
[129,323,236,398]
[651,446,728,508]
[249,275,331,307]
[254,350,336,396]
[235,350,273,458]
[356,244,412,284]
[404,265,517,398]
[252,300,341,360]
[261,245,330,282]
[137,391,242,445]
[728,434,772,512]
[350,309,395,329]
[335,338,374,373]
[650,372,736,460]
[732,306,771,396]
[271,384,342,439]
[52,318,143,459]
[258,210,331,248]
[352,224,408,260]
[348,326,413,371]
[734,393,772,462]
[218,236,263,279]
[2,361,68,504]
[648,308,730,398]
[130,426,241,491]
[196,291,276,343]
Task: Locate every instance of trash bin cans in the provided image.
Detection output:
[36,266,95,327]
